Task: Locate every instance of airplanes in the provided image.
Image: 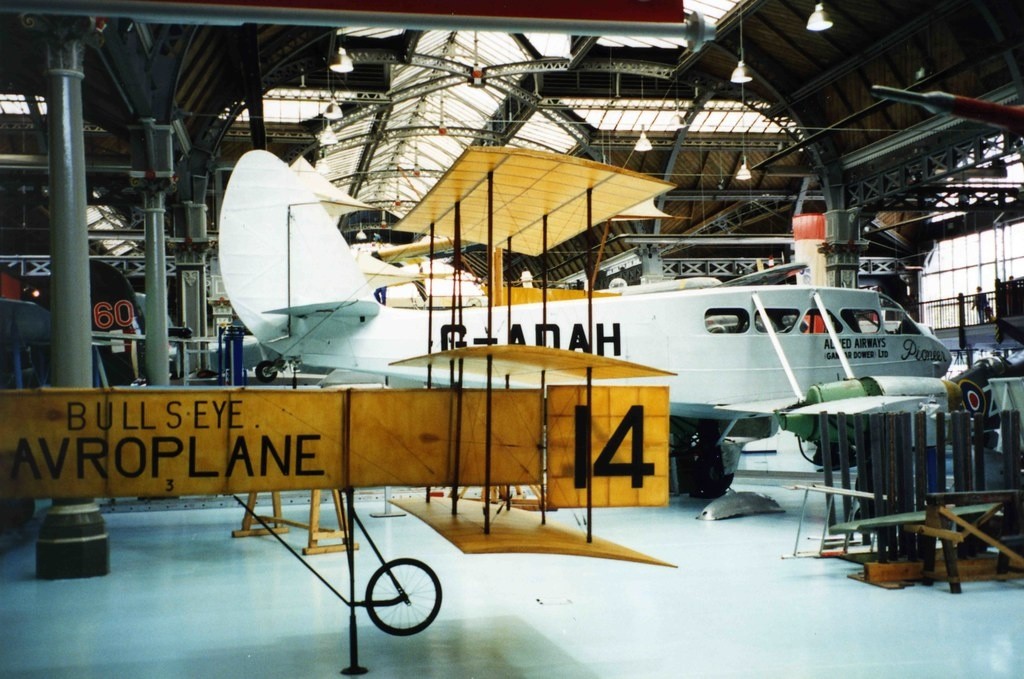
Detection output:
[0,261,295,533]
[214,143,954,500]
[773,314,1024,529]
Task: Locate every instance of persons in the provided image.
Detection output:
[971,287,991,323]
[1005,276,1015,304]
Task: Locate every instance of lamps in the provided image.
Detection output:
[318,70,339,144]
[323,71,343,119]
[329,27,354,72]
[671,72,687,128]
[736,83,752,180]
[806,3,834,31]
[730,0,753,83]
[633,75,653,152]
